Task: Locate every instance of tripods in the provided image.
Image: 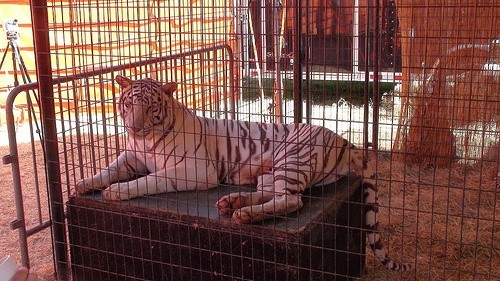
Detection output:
[1,31,31,87]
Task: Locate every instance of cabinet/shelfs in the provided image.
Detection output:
[65,173,366,281]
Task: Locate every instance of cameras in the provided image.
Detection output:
[5,19,18,30]
